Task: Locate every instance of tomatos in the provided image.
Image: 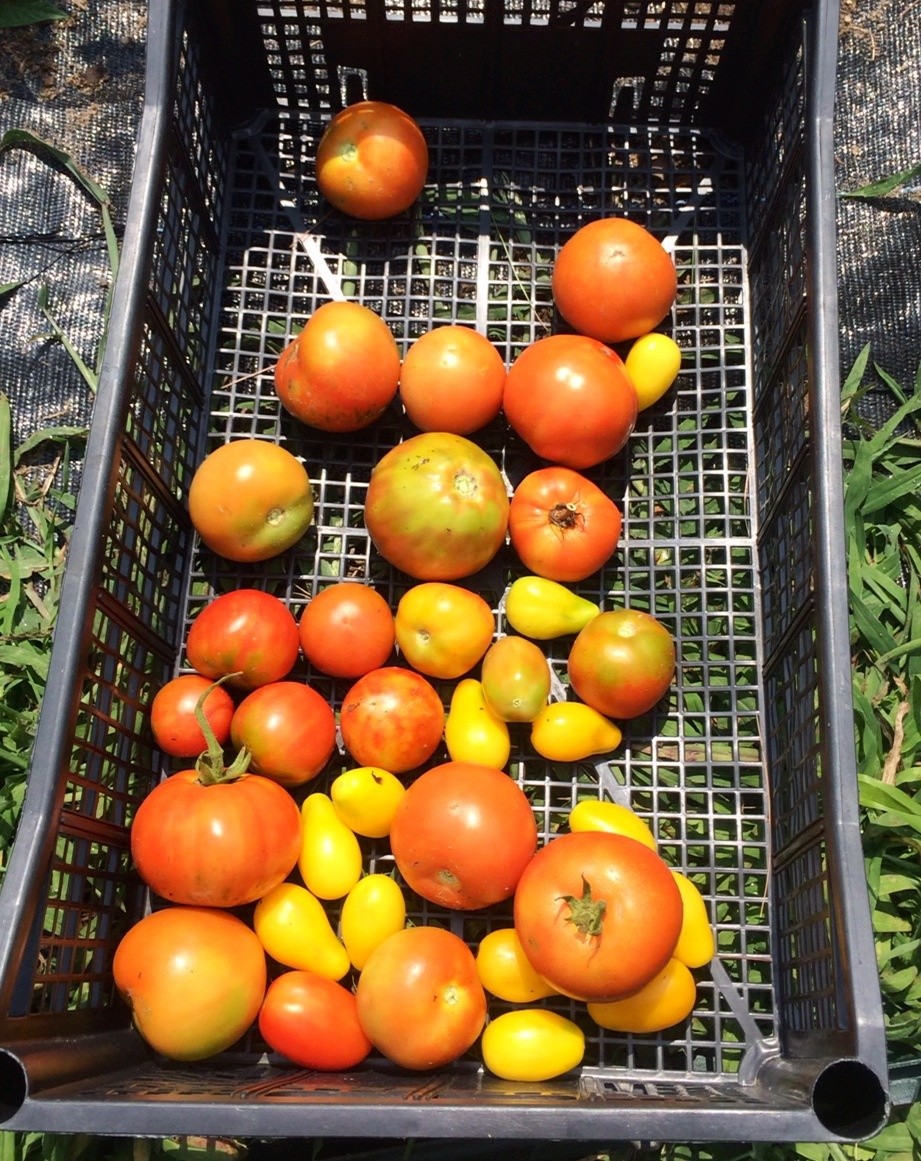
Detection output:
[112,216,717,1083]
[315,101,429,222]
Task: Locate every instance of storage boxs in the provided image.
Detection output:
[0,0,892,1143]
